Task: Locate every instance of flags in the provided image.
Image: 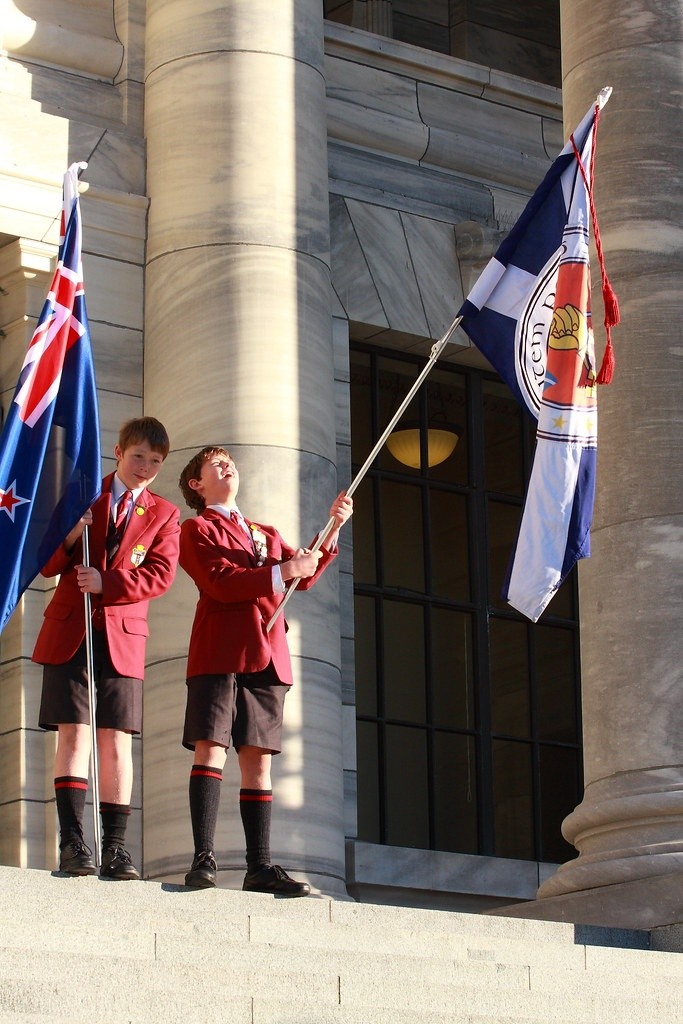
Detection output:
[0,167,102,632]
[455,108,600,624]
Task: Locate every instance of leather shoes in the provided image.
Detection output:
[185,850,217,887]
[59,842,97,875]
[99,848,139,880]
[242,865,310,897]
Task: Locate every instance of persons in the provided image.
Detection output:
[178,446,355,895]
[32,417,182,879]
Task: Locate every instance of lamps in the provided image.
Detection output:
[379,382,462,471]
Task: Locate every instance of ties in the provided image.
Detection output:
[229,510,254,554]
[93,491,133,632]
